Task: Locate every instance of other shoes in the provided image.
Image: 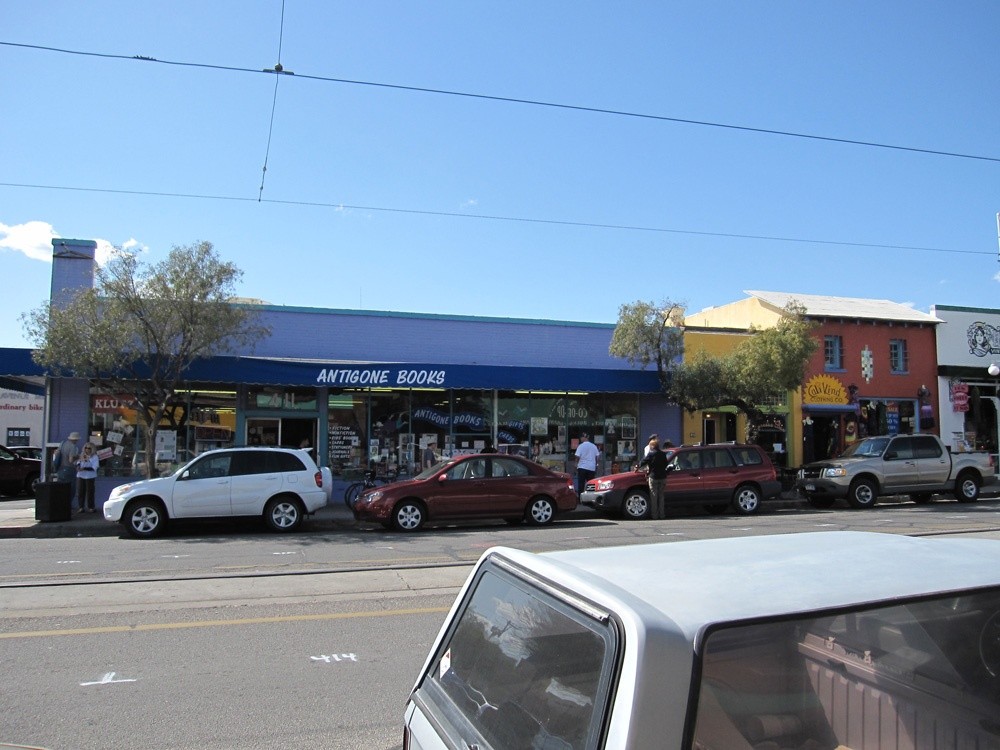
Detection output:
[77,508,84,513]
[88,508,98,512]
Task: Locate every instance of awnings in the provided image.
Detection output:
[0,347,686,393]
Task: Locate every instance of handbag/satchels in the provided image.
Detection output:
[52,441,66,469]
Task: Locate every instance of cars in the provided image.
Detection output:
[353,454,577,532]
[0,444,41,499]
[401,529,1000,750]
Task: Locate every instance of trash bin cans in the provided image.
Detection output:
[35,481,72,521]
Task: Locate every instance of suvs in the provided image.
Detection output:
[579,440,782,521]
[102,445,332,539]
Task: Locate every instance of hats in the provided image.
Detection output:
[579,432,589,438]
[67,432,81,440]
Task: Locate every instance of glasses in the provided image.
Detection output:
[85,447,91,449]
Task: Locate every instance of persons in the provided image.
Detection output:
[55,432,81,499]
[575,432,600,503]
[299,436,310,449]
[481,438,497,453]
[644,434,659,456]
[635,440,668,520]
[685,454,692,469]
[424,440,436,470]
[663,441,674,465]
[77,442,99,512]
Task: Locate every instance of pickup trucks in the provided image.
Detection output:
[795,432,998,509]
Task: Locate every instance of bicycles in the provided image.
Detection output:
[345,465,405,511]
